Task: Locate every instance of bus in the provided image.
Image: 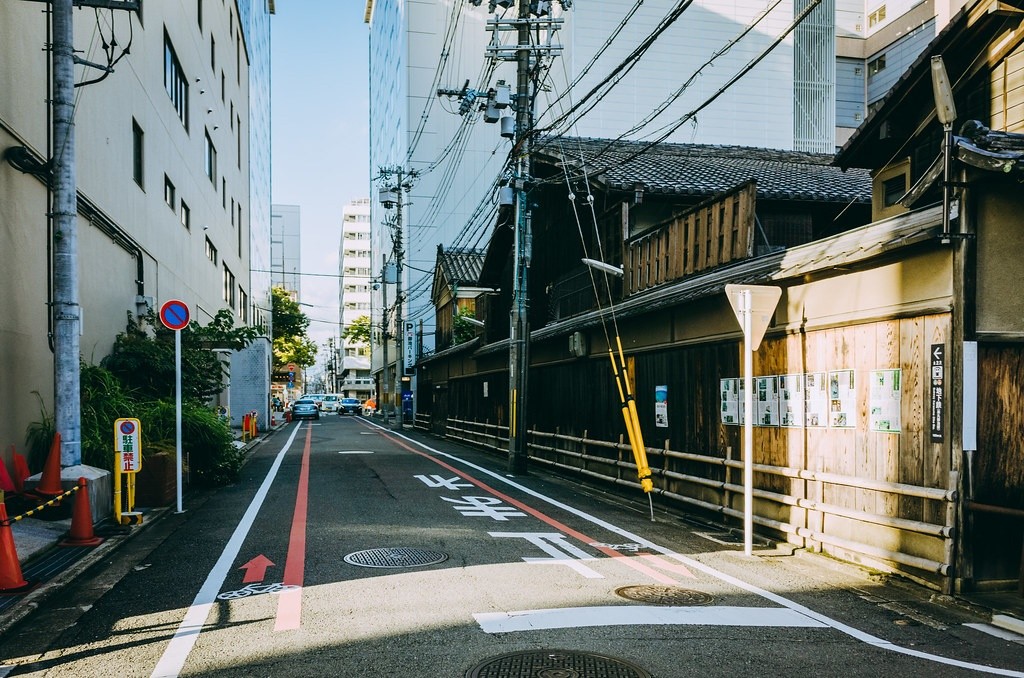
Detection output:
[300,394,344,412]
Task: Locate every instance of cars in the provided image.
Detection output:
[290,399,321,420]
[337,398,362,415]
[364,398,376,414]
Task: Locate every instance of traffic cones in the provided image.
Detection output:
[241,413,250,432]
[33,431,67,495]
[0,502,30,593]
[55,478,108,546]
[271,415,276,427]
